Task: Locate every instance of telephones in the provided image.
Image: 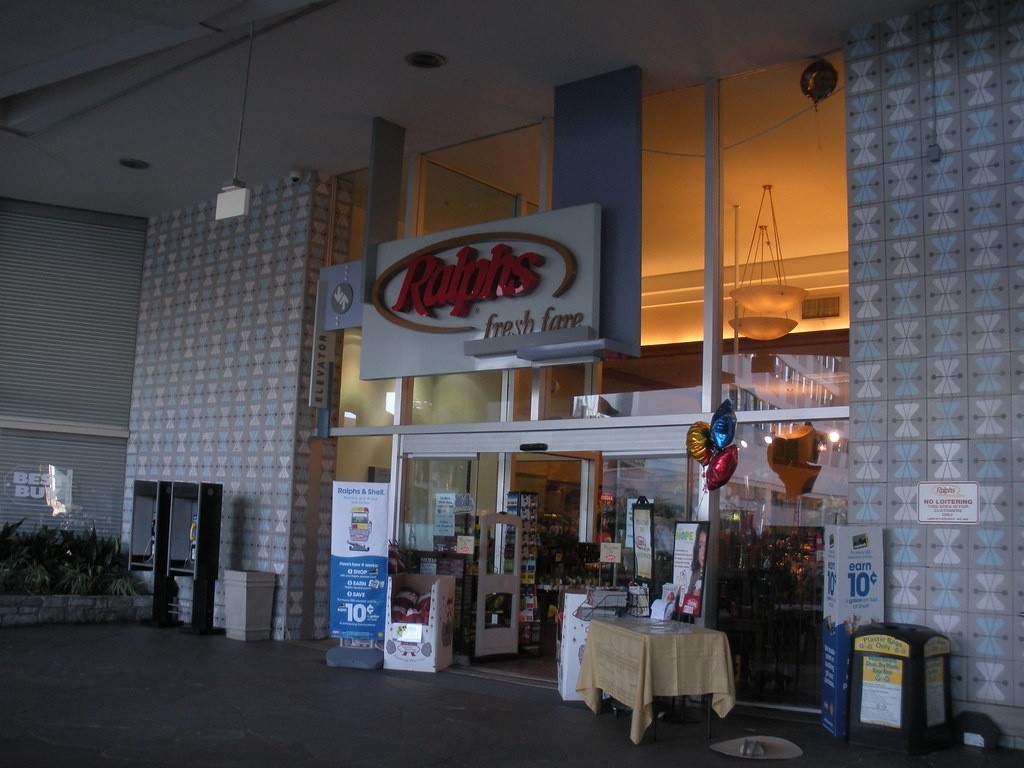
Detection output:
[150,511,156,543]
[188,520,197,564]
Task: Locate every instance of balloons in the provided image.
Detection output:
[686,398,741,493]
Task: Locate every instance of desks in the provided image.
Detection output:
[575,616,736,746]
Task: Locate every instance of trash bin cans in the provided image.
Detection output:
[841,621,956,756]
[224,569,276,642]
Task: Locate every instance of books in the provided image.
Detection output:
[576,587,627,621]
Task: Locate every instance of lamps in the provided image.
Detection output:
[764,431,775,443]
[730,183,809,315]
[740,437,752,448]
[728,225,798,342]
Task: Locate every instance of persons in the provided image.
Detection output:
[686,520,710,597]
